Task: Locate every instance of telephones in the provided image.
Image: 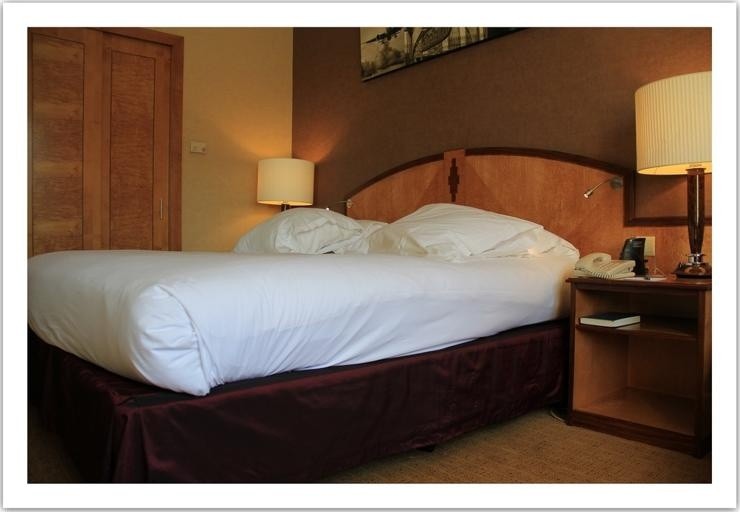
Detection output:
[574,252,636,280]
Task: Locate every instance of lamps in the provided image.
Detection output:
[634,71,712,278]
[257,159,315,213]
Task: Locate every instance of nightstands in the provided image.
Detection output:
[565,270,712,461]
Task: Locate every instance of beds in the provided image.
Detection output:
[28,146,635,483]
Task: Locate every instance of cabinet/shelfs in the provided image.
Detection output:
[27,27,184,254]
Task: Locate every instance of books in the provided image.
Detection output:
[579,311,641,329]
